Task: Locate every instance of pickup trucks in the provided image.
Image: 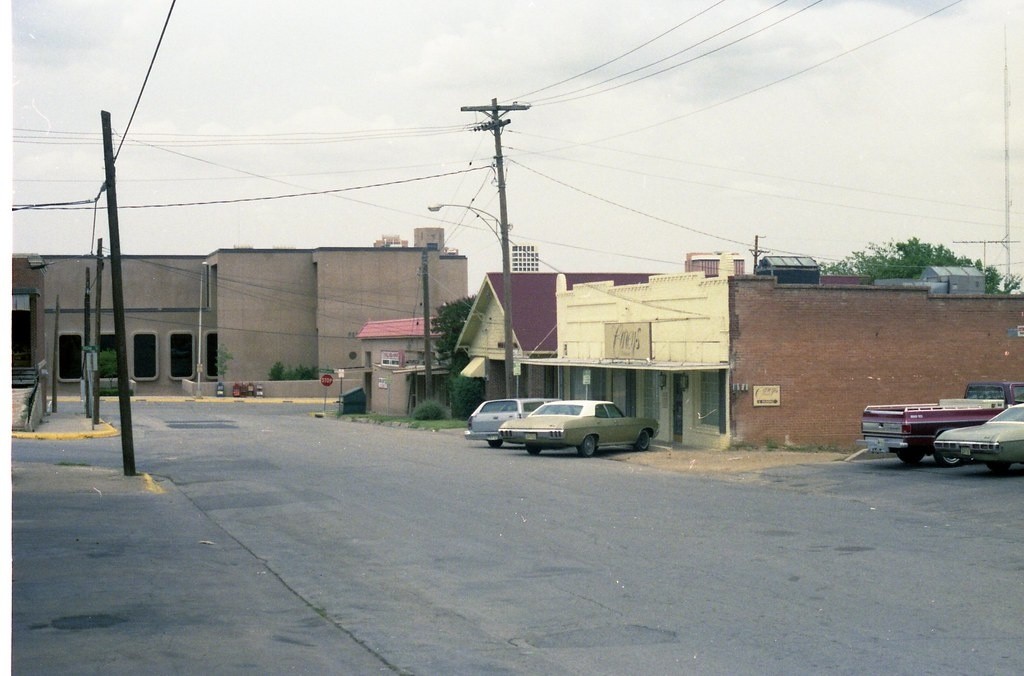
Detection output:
[855,381,1024,469]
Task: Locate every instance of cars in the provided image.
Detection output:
[934,401,1024,475]
[464,396,572,449]
[498,399,660,458]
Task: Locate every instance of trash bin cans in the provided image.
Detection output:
[233,381,263,397]
[215,381,224,396]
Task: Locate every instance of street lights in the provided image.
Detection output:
[195,260,210,399]
[426,202,514,399]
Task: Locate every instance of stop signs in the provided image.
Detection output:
[320,375,334,387]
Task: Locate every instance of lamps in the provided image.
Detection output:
[679,371,689,391]
[658,371,666,389]
